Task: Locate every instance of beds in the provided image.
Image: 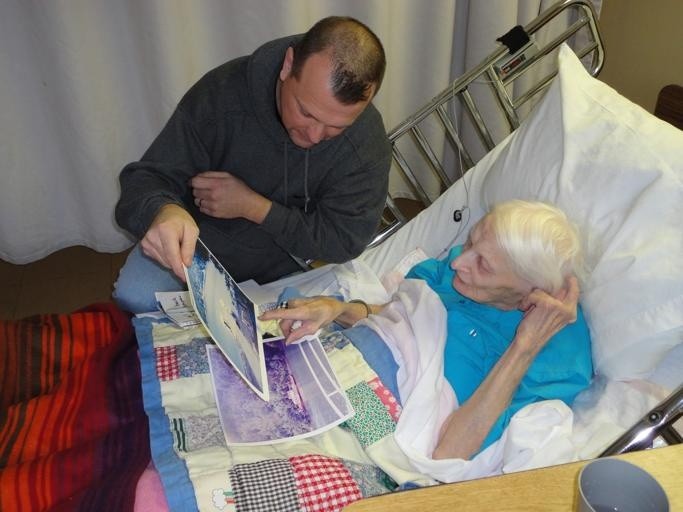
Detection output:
[0,84,683,512]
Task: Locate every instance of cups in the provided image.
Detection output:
[575,456,672,512]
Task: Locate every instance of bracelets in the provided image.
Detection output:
[349,299,372,319]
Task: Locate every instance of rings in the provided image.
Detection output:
[199,199,204,207]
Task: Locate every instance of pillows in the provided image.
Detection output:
[478,41,683,380]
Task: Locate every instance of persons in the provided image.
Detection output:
[257,197,593,461]
[111,17,392,314]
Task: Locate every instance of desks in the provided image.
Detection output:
[340,441,683,512]
